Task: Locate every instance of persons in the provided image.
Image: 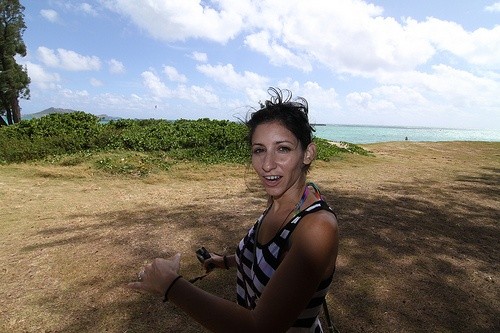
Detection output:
[128,87,338,333]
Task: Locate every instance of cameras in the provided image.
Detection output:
[196,247,216,270]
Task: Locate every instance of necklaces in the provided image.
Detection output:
[252,188,306,285]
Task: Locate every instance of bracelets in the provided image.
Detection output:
[164,275,183,302]
[223,255,229,268]
